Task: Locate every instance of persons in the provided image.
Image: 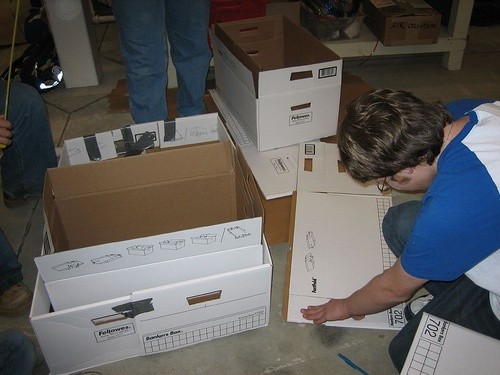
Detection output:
[300,89,500,375]
[100,0,211,125]
[0,79,58,317]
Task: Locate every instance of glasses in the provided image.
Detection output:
[377,177,391,192]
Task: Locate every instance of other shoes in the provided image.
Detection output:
[405,294,434,321]
[4,170,58,208]
[0,281,33,316]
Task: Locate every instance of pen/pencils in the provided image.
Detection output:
[338,353,373,375]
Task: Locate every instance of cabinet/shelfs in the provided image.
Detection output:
[167,0,474,89]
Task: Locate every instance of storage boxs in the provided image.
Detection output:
[29,0,500,375]
